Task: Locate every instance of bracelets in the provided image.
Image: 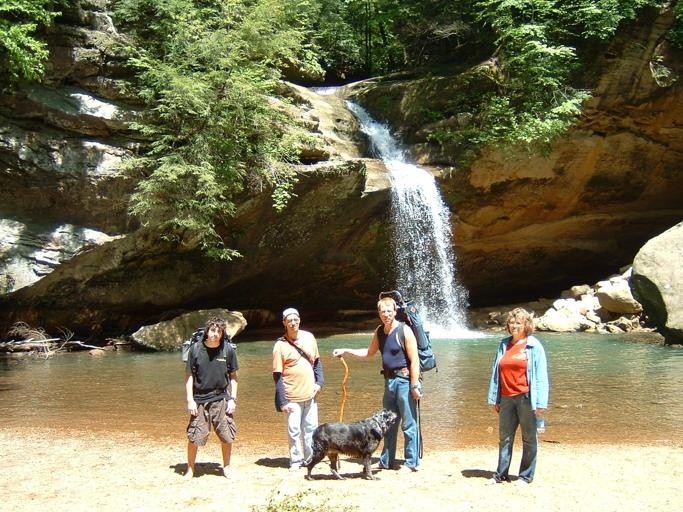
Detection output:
[230,397,238,401]
[411,384,418,389]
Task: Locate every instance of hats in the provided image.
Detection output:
[282,308,299,321]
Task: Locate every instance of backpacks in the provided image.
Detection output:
[376,290,438,374]
[187,328,230,386]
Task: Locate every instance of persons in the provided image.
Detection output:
[331,297,423,474]
[487,307,550,485]
[271,308,325,473]
[184,316,238,479]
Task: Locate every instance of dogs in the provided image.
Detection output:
[299,407,400,481]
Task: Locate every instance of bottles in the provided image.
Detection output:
[536,414,545,434]
